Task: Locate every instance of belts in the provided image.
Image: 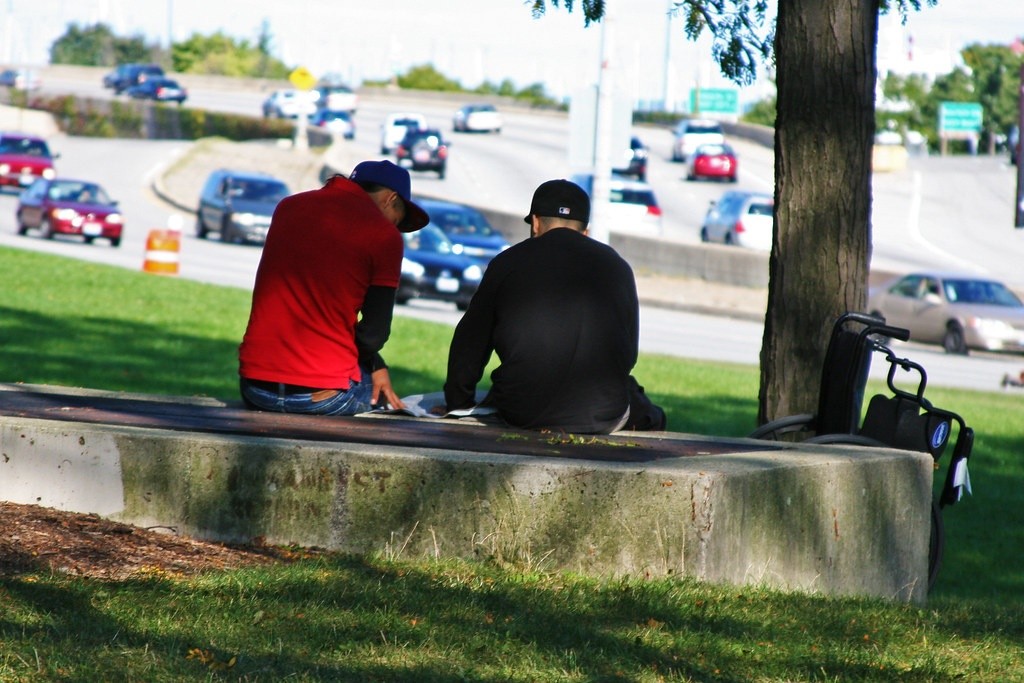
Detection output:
[246,378,323,394]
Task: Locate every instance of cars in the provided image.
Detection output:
[569,172,663,220]
[393,195,511,312]
[103,62,187,105]
[688,144,737,182]
[452,102,503,134]
[17,179,124,246]
[0,67,43,89]
[260,87,361,140]
[195,169,289,246]
[880,274,1024,356]
[0,133,61,190]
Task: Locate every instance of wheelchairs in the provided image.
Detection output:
[749,311,974,592]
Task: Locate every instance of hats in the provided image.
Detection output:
[348,159,431,233]
[524,178,591,224]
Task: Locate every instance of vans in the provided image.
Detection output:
[669,119,729,160]
[700,190,774,250]
[380,112,430,153]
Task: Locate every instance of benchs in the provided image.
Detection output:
[0,391,934,606]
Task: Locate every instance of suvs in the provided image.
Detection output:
[397,130,449,180]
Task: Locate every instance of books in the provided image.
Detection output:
[371,389,499,419]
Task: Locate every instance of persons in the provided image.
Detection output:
[238,160,431,416]
[427,178,667,436]
[72,190,92,203]
